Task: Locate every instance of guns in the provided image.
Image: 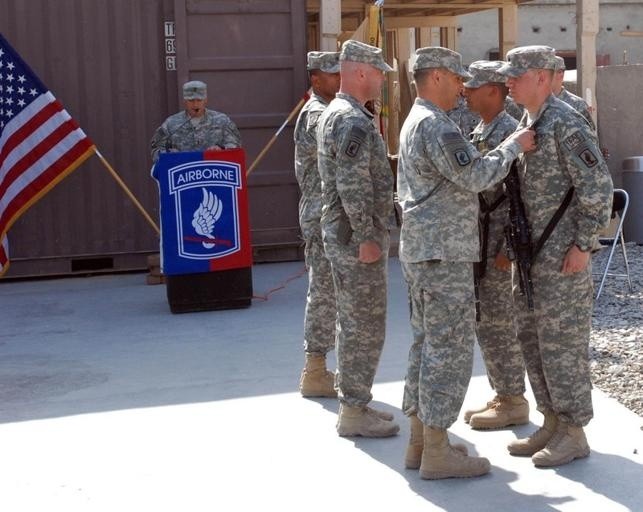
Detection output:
[504,160,535,312]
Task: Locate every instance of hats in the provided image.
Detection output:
[495,45,558,79]
[554,55,566,72]
[411,46,473,84]
[179,80,207,102]
[337,39,394,74]
[461,59,508,90]
[304,50,342,75]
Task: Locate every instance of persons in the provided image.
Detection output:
[316,42,399,439]
[447,94,524,142]
[548,53,595,129]
[451,49,598,432]
[395,45,539,481]
[293,49,348,398]
[504,45,616,466]
[149,80,242,165]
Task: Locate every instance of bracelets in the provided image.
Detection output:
[576,245,591,252]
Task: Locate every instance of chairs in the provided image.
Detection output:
[591,188,632,300]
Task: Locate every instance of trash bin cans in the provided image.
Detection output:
[622,155,643,246]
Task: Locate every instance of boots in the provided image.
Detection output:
[504,408,560,459]
[334,401,400,439]
[529,416,593,475]
[403,407,467,474]
[298,354,343,400]
[418,420,493,484]
[461,391,531,432]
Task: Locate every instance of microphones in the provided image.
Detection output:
[165,109,199,152]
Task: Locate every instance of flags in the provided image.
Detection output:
[0,34,98,281]
